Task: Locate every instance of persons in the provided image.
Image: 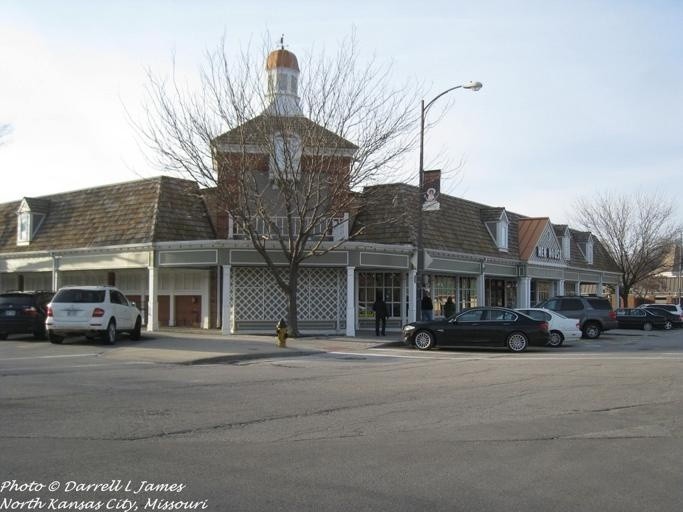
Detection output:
[373,295,389,336]
[444,296,456,317]
[421,292,433,320]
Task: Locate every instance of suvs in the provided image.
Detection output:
[532,293,617,339]
[0,285,142,344]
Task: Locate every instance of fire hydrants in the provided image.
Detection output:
[276,319,288,348]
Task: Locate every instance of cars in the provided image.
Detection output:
[615,303,682,331]
[400,305,551,353]
[497,308,583,347]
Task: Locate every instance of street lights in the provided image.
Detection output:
[416,81,483,322]
